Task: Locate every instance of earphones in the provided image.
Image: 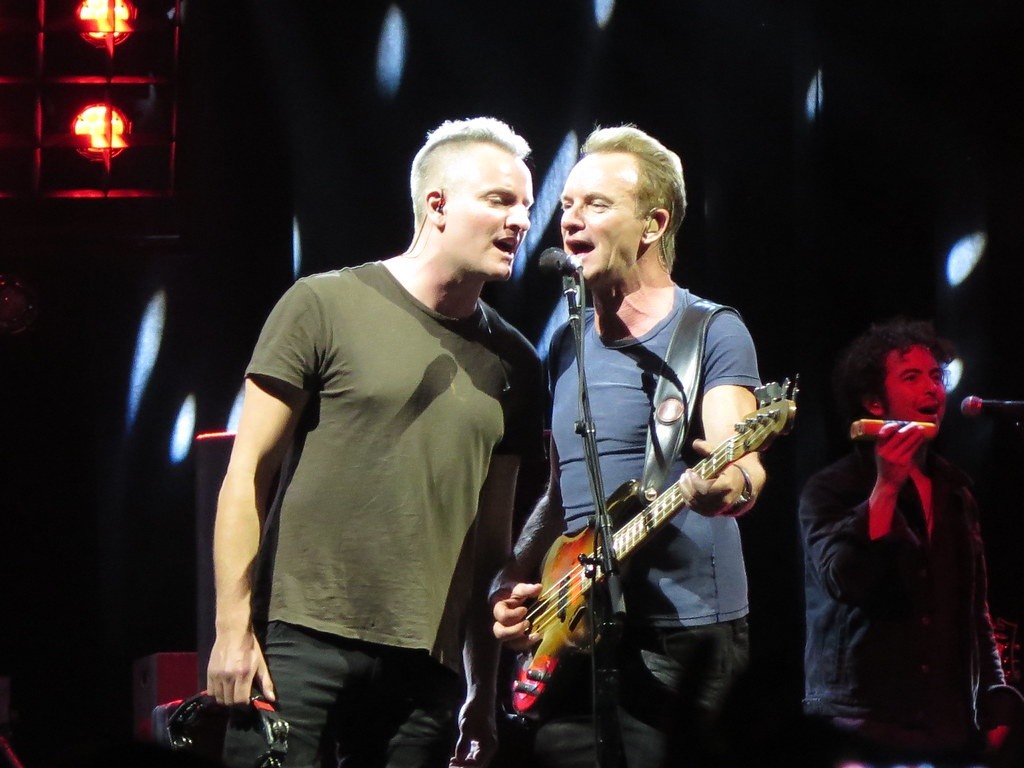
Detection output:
[434,199,444,215]
[648,218,659,233]
[872,401,880,408]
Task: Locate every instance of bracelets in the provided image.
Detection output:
[721,462,753,517]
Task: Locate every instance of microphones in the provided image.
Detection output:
[539,247,584,278]
[959,396,1024,417]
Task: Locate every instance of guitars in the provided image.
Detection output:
[511,378,800,718]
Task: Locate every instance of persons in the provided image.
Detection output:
[202,117,555,768]
[484,121,767,768]
[796,321,1024,768]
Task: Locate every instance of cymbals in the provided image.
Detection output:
[164,680,289,768]
